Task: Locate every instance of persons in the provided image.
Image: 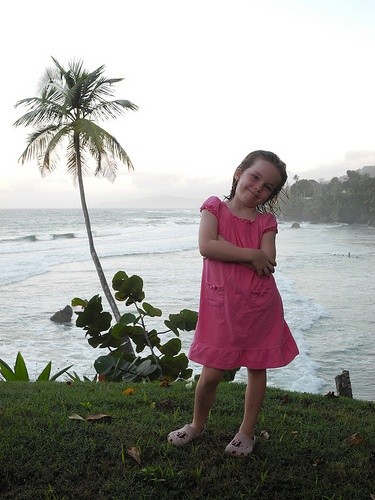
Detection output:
[166,149,300,456]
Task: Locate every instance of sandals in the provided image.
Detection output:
[166,423,207,447]
[224,432,257,458]
